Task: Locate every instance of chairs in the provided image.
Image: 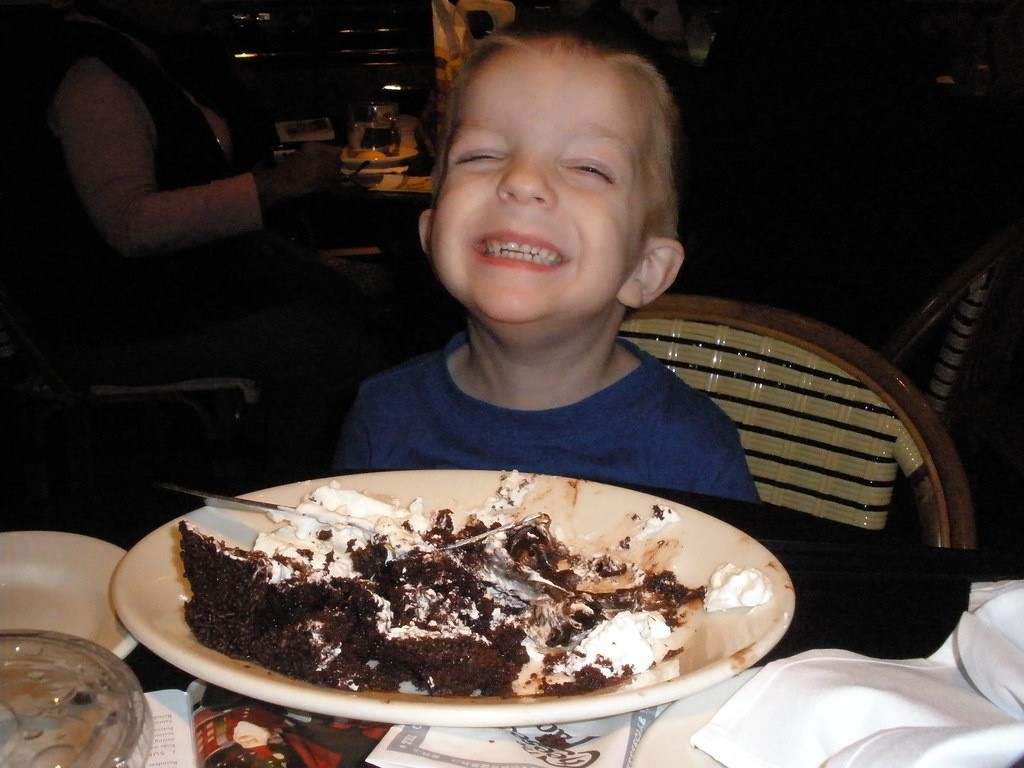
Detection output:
[0,284,256,410]
[618,220,1024,549]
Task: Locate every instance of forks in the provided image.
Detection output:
[152,478,538,556]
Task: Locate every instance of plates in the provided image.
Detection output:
[340,145,419,166]
[108,468,796,728]
[0,629,154,768]
[0,531,141,660]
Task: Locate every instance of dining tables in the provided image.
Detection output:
[0,422,1024,703]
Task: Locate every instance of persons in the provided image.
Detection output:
[331,32,763,503]
[42,0,366,473]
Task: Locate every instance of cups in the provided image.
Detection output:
[346,101,401,168]
